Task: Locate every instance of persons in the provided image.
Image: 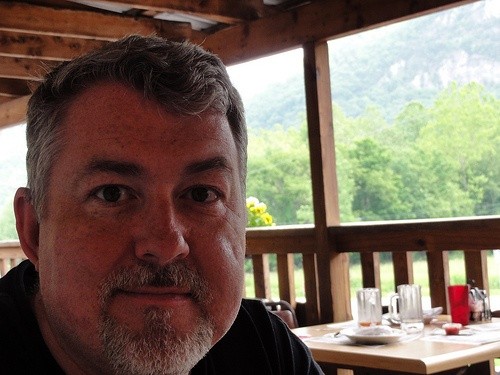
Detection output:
[0,33,327,375]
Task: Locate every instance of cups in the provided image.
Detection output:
[447,284,469,326]
[389,284,424,332]
[350,288,382,328]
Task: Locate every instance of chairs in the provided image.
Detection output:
[261,300,298,328]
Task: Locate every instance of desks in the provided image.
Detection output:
[289,312,500,375]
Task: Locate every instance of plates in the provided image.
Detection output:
[340,327,403,341]
